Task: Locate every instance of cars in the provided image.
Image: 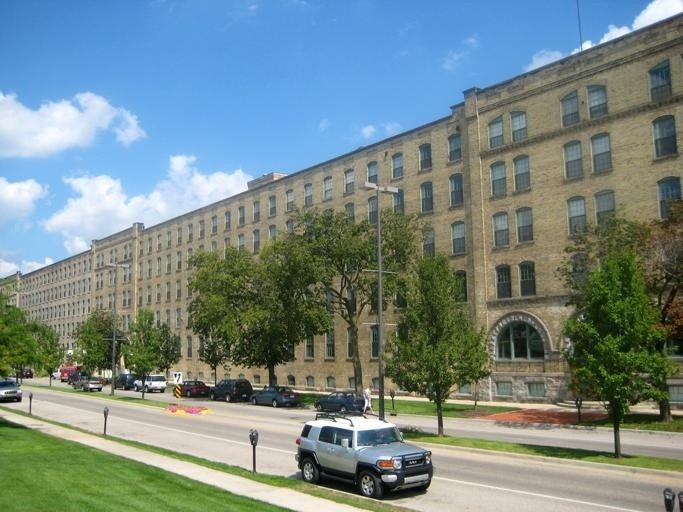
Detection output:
[0,377,22,403]
[52,366,102,393]
[313,390,372,414]
[17,369,32,378]
[249,385,305,408]
[172,380,211,398]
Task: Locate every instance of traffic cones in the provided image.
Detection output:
[175,384,182,399]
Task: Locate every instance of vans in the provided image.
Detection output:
[209,378,254,402]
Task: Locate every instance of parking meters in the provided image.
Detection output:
[248,429,258,473]
[28,393,32,414]
[103,408,108,435]
[575,397,581,423]
[663,488,674,512]
[389,389,395,410]
[677,492,682,512]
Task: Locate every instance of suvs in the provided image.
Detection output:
[133,374,167,393]
[115,373,138,390]
[294,412,434,500]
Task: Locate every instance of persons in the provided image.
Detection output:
[362,383,375,415]
